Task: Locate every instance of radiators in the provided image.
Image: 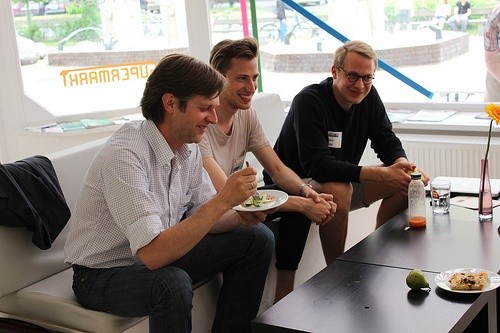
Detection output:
[360,134,500,182]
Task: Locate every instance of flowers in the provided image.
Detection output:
[478,104,500,213]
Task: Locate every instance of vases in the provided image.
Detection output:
[478,159,494,220]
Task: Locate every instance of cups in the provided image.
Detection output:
[429,181,450,214]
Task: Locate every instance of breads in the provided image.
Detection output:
[449,272,488,290]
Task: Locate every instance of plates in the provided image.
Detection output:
[231,190,289,212]
[434,268,500,293]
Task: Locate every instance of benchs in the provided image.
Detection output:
[210,14,325,32]
[409,7,494,33]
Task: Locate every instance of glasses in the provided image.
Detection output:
[339,66,375,84]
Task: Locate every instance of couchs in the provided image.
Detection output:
[0,93,383,332]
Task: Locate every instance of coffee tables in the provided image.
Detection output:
[335,195,500,274]
[251,260,500,333]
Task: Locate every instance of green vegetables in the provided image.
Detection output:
[245,195,263,207]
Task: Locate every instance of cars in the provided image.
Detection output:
[44,7,66,15]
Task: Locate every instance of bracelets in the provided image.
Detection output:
[299,183,313,197]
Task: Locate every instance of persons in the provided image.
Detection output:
[64,53,274,333]
[483,7,500,102]
[263,40,429,265]
[448,0,471,32]
[394,0,415,30]
[432,0,451,30]
[197,36,336,303]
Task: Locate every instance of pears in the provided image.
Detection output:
[405,268,431,289]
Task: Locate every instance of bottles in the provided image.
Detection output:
[408,173,427,228]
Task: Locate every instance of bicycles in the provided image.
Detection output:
[258,11,315,44]
